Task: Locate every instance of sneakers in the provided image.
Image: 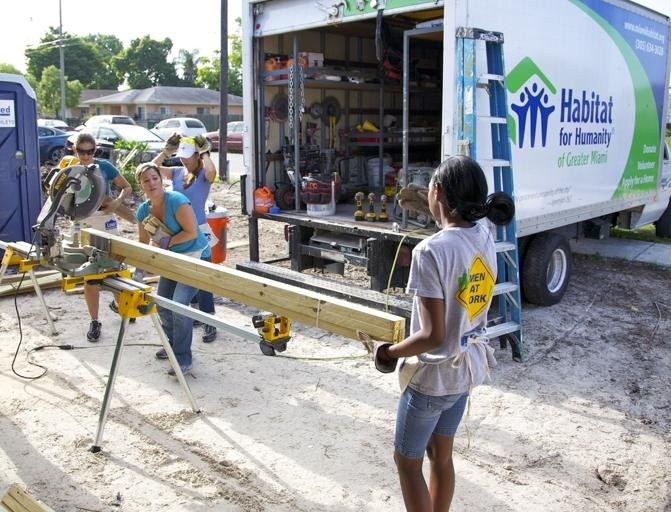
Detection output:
[108,298,138,324]
[193,318,219,344]
[166,359,195,379]
[86,320,104,344]
[154,347,170,362]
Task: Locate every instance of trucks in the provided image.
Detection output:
[242,0,671,308]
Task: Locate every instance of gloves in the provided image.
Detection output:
[193,134,210,156]
[374,337,398,376]
[396,181,434,219]
[162,132,184,160]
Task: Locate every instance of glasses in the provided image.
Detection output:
[75,147,97,157]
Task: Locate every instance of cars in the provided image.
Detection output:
[37,115,245,170]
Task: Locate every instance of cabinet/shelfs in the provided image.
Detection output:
[258,30,439,213]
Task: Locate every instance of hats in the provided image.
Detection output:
[174,136,199,160]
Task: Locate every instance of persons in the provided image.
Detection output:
[375,159,498,512]
[151,133,217,344]
[71,132,136,342]
[135,162,208,377]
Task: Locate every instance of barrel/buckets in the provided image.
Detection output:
[287,53,308,68]
[367,156,394,187]
[254,186,275,213]
[280,59,288,79]
[264,57,280,81]
[206,207,230,263]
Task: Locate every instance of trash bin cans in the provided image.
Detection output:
[202,204,232,262]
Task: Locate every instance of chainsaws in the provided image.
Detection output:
[36,155,79,224]
